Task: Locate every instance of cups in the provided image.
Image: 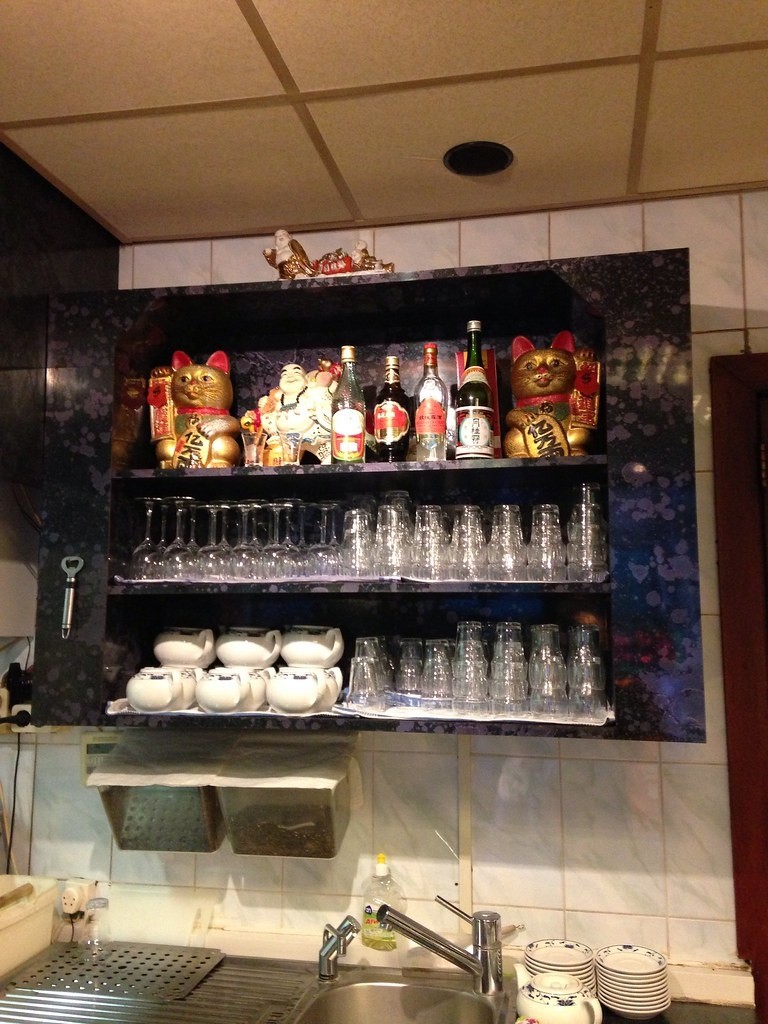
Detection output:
[278,431,304,466]
[241,431,268,468]
[340,480,607,581]
[83,897,113,964]
[346,620,606,716]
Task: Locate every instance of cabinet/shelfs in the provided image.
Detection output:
[101,447,615,742]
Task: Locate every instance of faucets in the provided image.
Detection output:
[375,894,504,996]
[319,915,362,980]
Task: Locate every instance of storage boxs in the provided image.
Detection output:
[0,873,58,976]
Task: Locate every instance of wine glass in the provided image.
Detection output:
[130,496,340,581]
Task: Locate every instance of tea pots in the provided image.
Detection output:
[215,626,283,667]
[125,667,207,711]
[280,625,344,668]
[151,625,217,667]
[513,963,602,1024]
[258,666,343,714]
[192,667,276,713]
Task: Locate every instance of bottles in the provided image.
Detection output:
[373,355,411,463]
[330,346,367,464]
[413,343,448,462]
[454,320,495,460]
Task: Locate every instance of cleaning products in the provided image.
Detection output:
[361,855,408,952]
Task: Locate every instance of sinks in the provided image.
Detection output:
[294,982,496,1024]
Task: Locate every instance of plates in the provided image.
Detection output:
[594,944,671,1019]
[523,938,597,997]
